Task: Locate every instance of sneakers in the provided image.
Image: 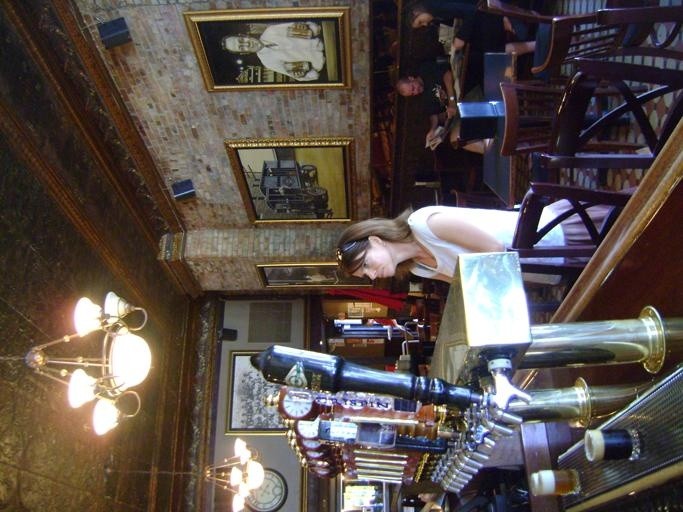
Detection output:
[520,142,532,171]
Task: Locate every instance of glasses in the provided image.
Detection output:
[335,241,357,266]
